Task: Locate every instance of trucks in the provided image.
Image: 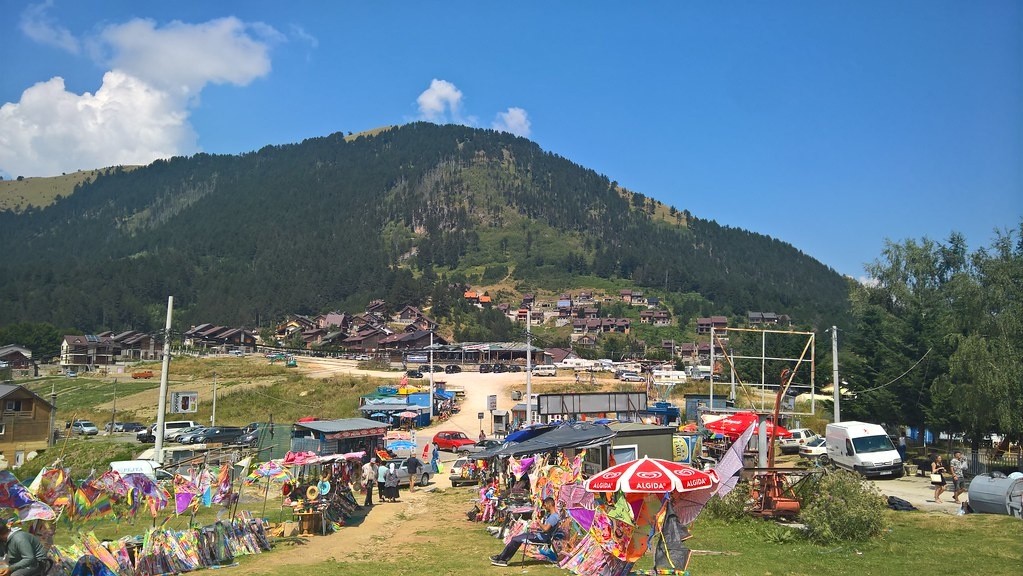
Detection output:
[132,370,154,379]
[136,420,198,443]
[826,421,904,479]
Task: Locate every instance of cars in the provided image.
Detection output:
[479,363,537,373]
[418,365,430,373]
[376,459,434,487]
[458,439,507,456]
[778,428,819,455]
[105,421,124,432]
[428,365,444,373]
[406,370,423,378]
[339,354,374,361]
[167,421,292,447]
[432,431,476,453]
[799,438,829,466]
[449,456,478,487]
[445,365,461,374]
[614,370,645,382]
[66,371,77,377]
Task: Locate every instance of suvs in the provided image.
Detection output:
[122,422,147,432]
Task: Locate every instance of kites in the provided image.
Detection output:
[0,452,299,576]
[459,447,694,576]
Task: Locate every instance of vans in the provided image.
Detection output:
[532,365,556,376]
[64,418,98,435]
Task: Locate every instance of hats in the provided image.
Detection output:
[371,458,376,463]
[283,469,331,500]
[411,454,416,457]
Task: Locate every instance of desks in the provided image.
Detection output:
[293,512,324,535]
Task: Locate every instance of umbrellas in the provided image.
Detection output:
[372,412,386,421]
[586,455,720,517]
[387,439,416,456]
[704,412,759,450]
[421,441,429,465]
[751,418,792,444]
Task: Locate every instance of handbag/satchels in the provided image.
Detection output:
[930,470,942,482]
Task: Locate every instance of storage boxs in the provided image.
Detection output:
[264,522,299,537]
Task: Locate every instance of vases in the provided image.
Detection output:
[904,465,919,474]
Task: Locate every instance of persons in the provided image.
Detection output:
[591,370,595,382]
[361,457,400,507]
[507,472,515,488]
[389,415,393,431]
[929,454,947,504]
[479,430,486,442]
[949,451,966,504]
[482,477,494,523]
[407,452,422,493]
[436,397,451,422]
[767,437,770,460]
[147,426,152,443]
[575,373,580,383]
[431,446,439,472]
[899,431,907,461]
[0,517,48,576]
[489,497,561,567]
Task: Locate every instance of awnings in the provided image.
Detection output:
[469,433,518,461]
[498,422,611,459]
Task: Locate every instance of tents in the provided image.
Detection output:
[398,411,419,429]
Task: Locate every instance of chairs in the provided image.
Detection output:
[522,522,560,568]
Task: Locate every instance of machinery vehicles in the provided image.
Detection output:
[750,368,803,520]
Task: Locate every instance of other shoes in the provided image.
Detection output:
[389,500,392,502]
[489,555,499,561]
[935,500,941,504]
[491,560,507,566]
[382,497,386,501]
[379,499,383,502]
[393,498,395,502]
[953,496,956,499]
[938,499,943,503]
[955,500,961,504]
